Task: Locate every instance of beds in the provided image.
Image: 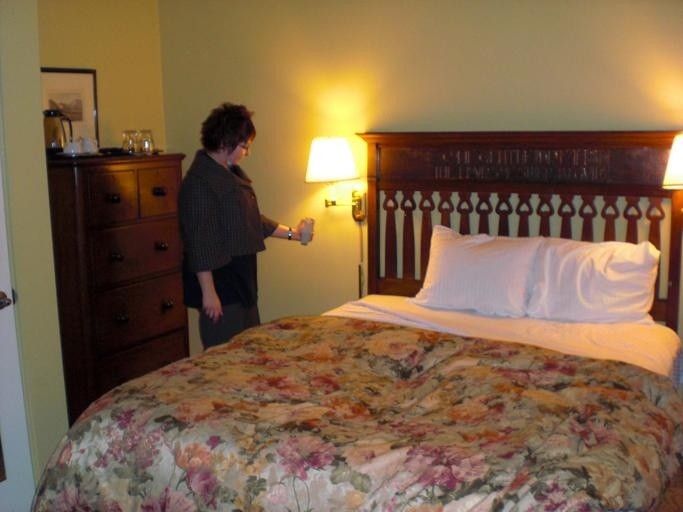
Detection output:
[30,131,683,512]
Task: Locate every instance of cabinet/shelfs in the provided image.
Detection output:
[46,153,190,410]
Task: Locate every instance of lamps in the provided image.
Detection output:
[662,131,683,192]
[304,137,367,263]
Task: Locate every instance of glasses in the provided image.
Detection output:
[241,143,248,149]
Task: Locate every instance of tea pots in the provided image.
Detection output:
[43,108,73,154]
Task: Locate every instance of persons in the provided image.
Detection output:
[177,103,313,353]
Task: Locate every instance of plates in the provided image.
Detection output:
[56,153,105,159]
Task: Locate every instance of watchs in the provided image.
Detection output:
[285,226,293,243]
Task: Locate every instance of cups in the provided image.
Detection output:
[139,130,156,154]
[60,136,102,155]
[119,130,137,155]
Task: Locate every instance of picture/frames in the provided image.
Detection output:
[42,66,99,157]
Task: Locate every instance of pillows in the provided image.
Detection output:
[412,225,541,319]
[526,237,662,328]
